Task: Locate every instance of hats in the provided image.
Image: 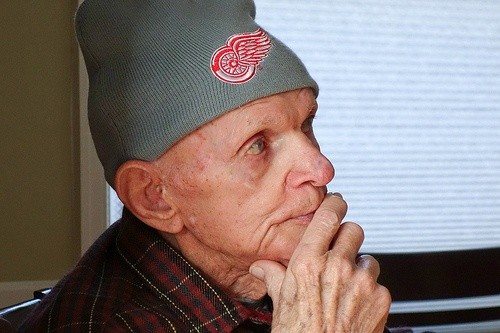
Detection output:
[75,0,319,189]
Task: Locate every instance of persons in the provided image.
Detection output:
[16,0,392,333]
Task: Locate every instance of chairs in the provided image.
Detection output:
[357,247,500,333]
